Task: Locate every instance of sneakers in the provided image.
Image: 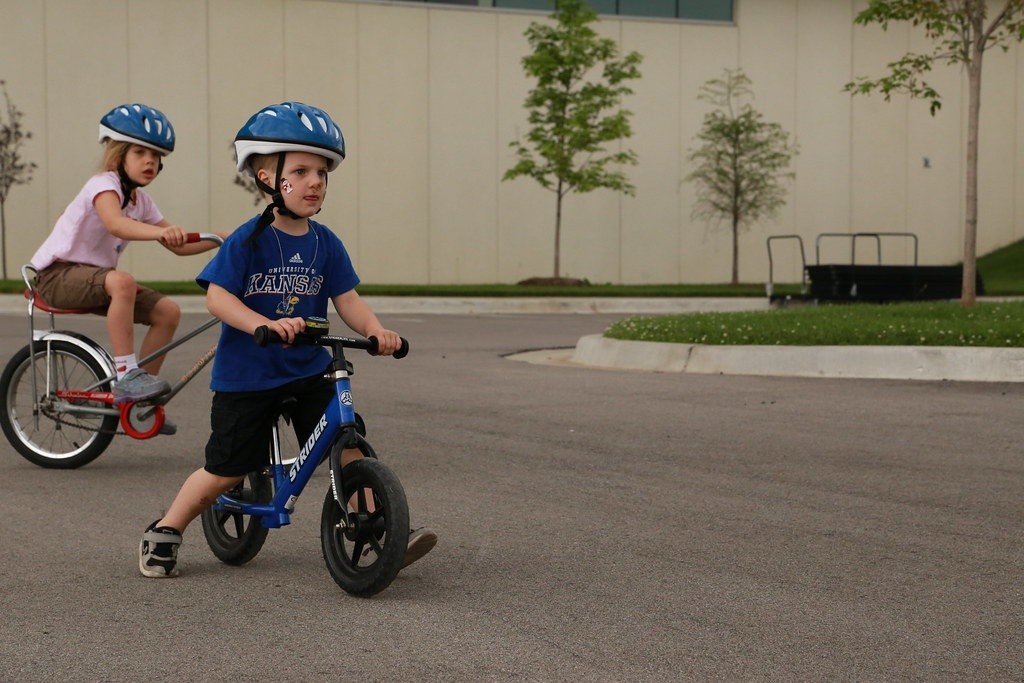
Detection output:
[157,419,177,436]
[400,526,438,570]
[139,518,183,578]
[113,369,171,403]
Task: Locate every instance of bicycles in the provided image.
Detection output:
[0,229,226,470]
[201,315,410,598]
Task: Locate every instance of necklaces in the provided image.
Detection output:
[271,223,319,315]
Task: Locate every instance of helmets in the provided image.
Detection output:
[98,103,175,156]
[234,101,345,172]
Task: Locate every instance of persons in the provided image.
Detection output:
[32,103,231,435]
[138,100,437,579]
